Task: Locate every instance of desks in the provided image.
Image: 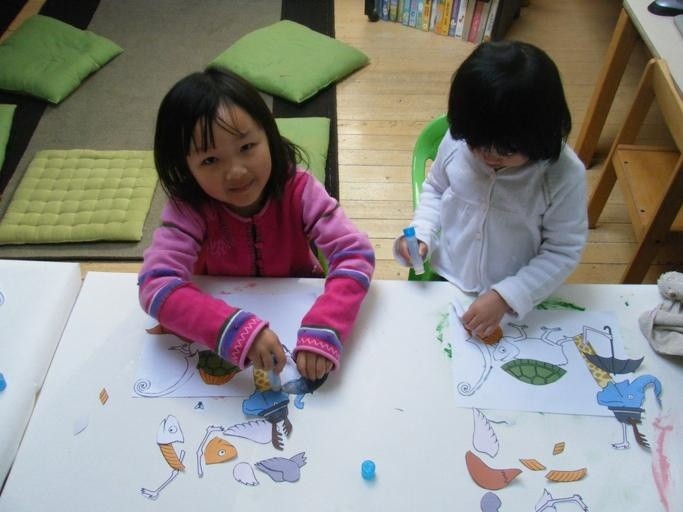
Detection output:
[573,0,683,171]
[1,270,683,512]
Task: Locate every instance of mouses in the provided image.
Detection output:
[648,0,683,17]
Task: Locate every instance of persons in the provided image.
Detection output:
[393,38,589,340]
[138,66,376,382]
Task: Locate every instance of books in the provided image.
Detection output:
[375,0,498,44]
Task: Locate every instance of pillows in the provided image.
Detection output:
[198,15,368,99]
[1,103,15,168]
[270,112,330,192]
[0,14,123,106]
[1,145,162,240]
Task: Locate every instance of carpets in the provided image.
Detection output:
[2,0,341,280]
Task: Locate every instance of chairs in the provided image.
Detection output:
[405,113,449,281]
[587,56,683,285]
